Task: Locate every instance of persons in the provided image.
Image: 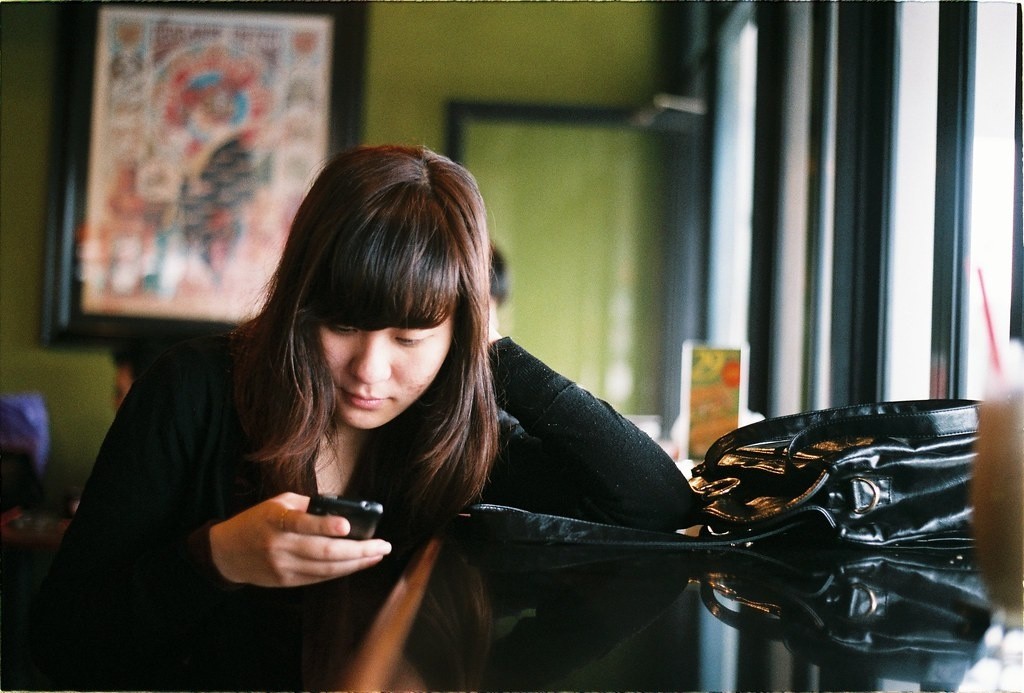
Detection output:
[113,339,168,411]
[23,144,693,623]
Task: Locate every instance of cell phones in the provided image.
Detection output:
[306,492,382,542]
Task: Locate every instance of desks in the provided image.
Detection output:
[335,537,993,693]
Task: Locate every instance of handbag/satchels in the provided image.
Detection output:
[459,550,993,686]
[475,398,985,551]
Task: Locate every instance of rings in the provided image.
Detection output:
[279,508,289,530]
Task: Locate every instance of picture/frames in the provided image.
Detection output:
[39,0,366,349]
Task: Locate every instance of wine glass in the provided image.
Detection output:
[968,336,1024,693]
